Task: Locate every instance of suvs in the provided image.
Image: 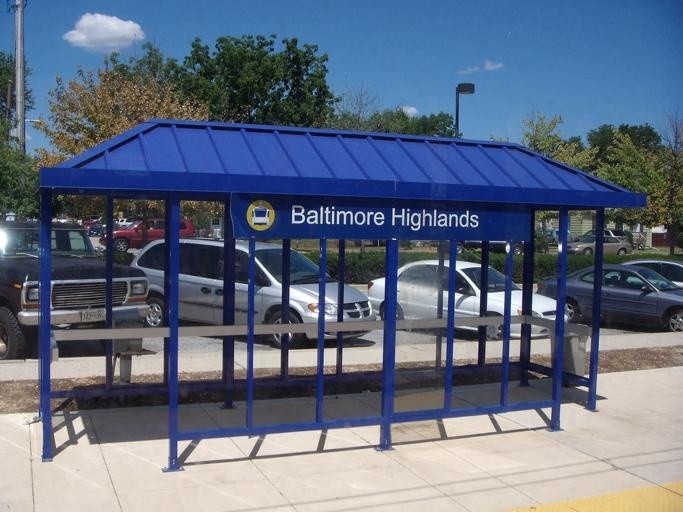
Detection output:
[0,220,151,360]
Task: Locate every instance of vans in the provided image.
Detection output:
[128,238,376,351]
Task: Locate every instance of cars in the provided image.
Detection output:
[576,228,630,243]
[99,218,194,253]
[537,262,683,331]
[366,259,572,341]
[4,211,142,238]
[567,235,634,257]
[617,258,683,291]
[462,240,525,256]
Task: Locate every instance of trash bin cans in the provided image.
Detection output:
[214,229,224,241]
[550,324,591,386]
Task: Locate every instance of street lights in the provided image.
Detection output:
[452,82,476,140]
